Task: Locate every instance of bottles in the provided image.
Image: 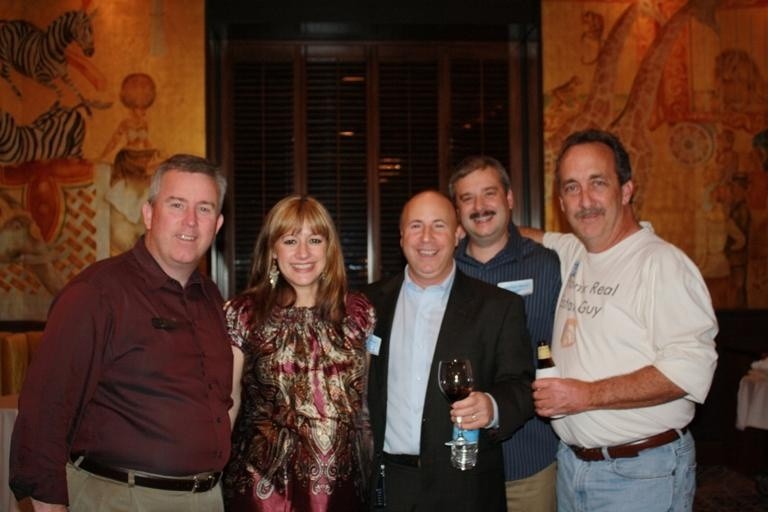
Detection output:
[536,340,567,419]
[450,427,479,471]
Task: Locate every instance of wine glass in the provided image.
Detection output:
[438,358,477,447]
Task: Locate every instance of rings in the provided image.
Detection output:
[472,414,480,422]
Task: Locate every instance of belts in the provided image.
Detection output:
[570,429,684,460]
[72,454,220,494]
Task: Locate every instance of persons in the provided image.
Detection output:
[720,172,753,310]
[448,153,563,512]
[8,152,238,512]
[454,128,719,511]
[752,128,768,173]
[351,189,534,512]
[219,193,380,512]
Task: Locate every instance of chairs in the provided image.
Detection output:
[0,330,43,512]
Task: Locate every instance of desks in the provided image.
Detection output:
[736,354,768,437]
